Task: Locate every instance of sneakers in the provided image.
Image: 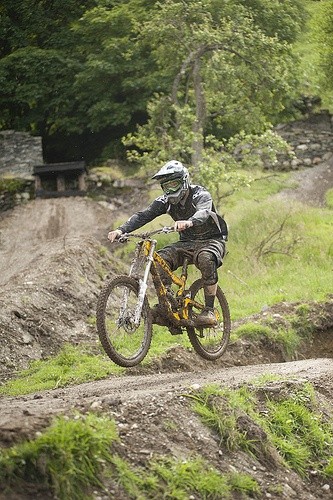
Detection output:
[142,304,171,322]
[196,307,217,325]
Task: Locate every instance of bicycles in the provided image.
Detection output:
[95,223,232,368]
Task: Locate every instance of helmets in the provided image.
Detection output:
[151,160,190,205]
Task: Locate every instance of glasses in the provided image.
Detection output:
[161,172,189,194]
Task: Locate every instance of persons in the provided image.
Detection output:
[108,160,228,326]
